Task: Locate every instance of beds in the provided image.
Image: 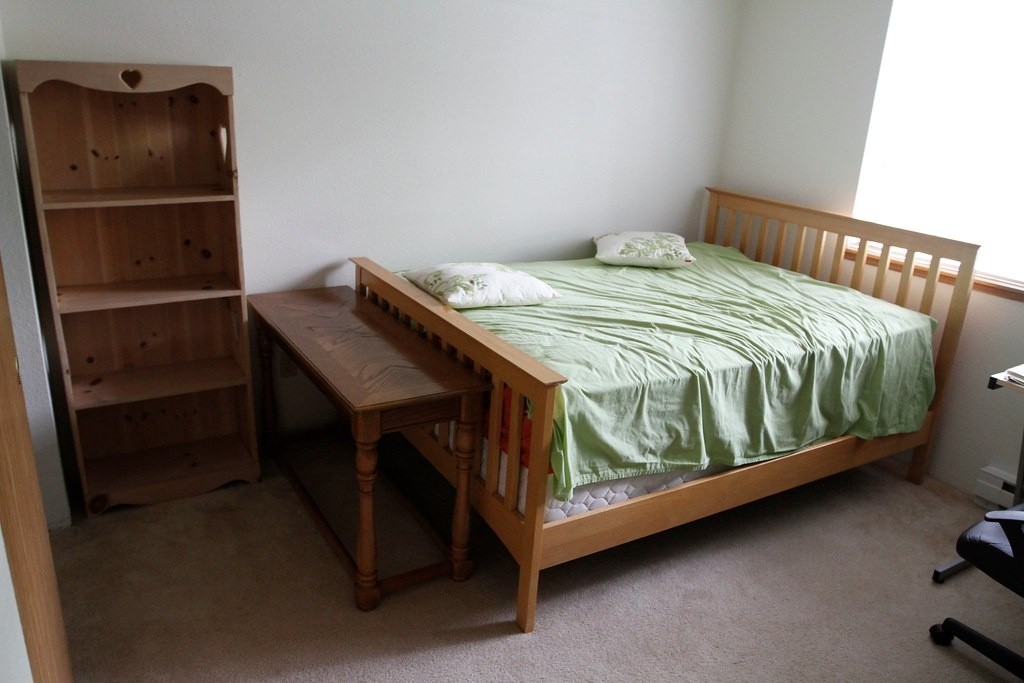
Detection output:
[348,186,984,634]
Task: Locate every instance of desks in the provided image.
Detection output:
[931,364,1024,585]
[245,285,496,614]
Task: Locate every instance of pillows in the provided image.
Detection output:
[403,262,564,310]
[593,231,697,268]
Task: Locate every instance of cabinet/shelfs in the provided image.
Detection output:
[0,58,264,522]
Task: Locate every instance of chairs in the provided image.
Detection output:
[929,503,1024,680]
